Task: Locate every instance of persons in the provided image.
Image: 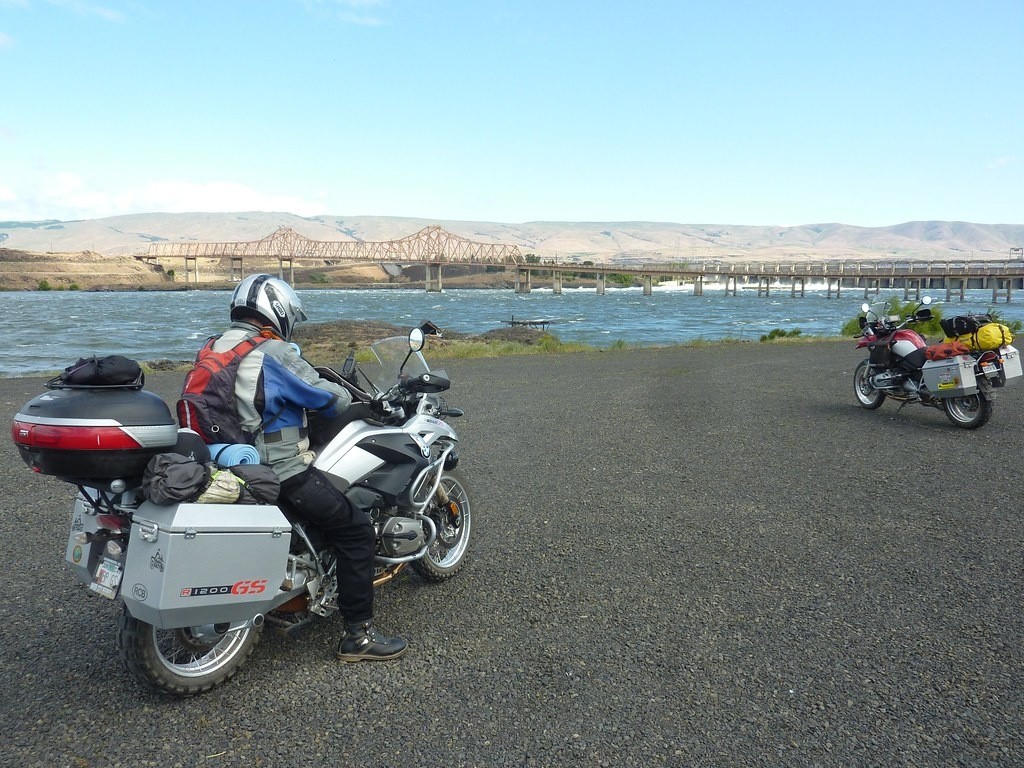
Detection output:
[174,274,409,663]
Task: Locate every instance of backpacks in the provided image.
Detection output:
[176,334,288,447]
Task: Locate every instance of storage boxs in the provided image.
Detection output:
[120,499,292,630]
[992,345,1023,379]
[11,390,176,495]
[921,355,978,399]
[66,487,136,595]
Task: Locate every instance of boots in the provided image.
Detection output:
[335,617,409,663]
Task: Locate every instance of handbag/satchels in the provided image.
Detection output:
[867,340,890,364]
[939,313,993,338]
[141,452,280,506]
[925,341,970,361]
[939,323,1016,351]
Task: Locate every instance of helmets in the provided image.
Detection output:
[229,272,308,341]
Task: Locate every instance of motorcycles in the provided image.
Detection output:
[852,296,1024,430]
[9,326,476,702]
